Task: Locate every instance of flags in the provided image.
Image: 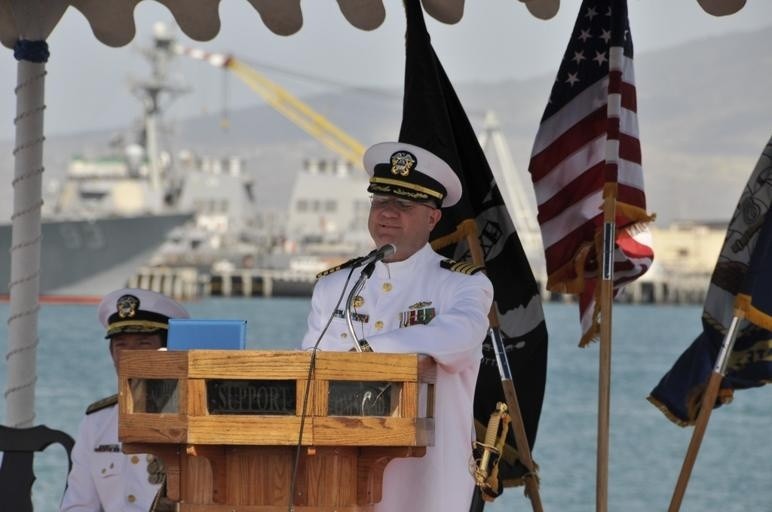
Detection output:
[399,0,548,512]
[644,135,770,429]
[529,0,653,347]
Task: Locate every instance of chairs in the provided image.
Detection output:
[0,422,78,512]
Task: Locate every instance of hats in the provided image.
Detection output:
[363,142,463,209]
[96,288,192,339]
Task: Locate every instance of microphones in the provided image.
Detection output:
[355,244,397,267]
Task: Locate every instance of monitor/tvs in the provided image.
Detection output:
[167,319,247,350]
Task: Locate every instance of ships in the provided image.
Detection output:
[61,20,549,303]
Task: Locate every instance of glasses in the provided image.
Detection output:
[370,195,436,210]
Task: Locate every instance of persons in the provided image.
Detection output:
[303,141,493,512]
[57,287,189,511]
